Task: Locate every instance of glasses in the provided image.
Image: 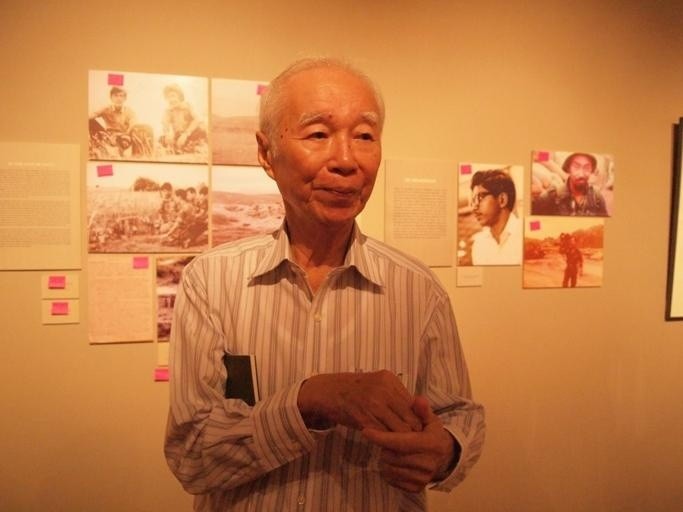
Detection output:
[470,191,490,207]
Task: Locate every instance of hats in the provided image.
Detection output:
[562,153,597,173]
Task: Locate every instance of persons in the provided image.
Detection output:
[470,150,611,288]
[163,54,486,512]
[88,82,211,252]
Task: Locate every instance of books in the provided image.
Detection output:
[222,355,263,405]
